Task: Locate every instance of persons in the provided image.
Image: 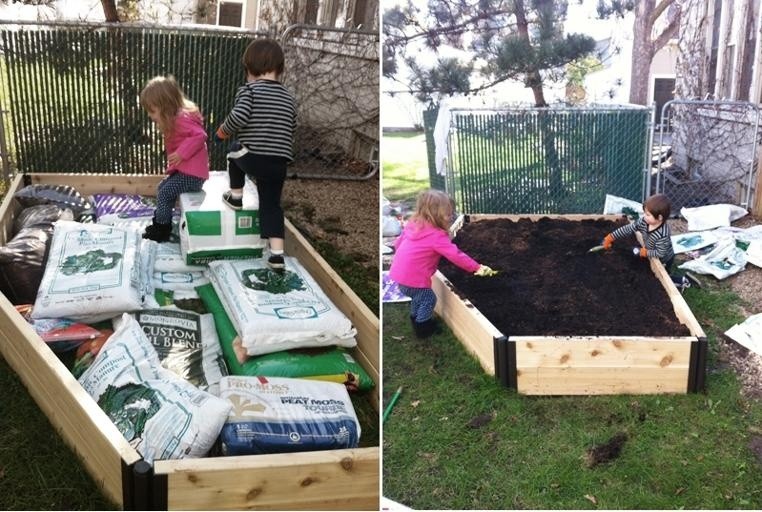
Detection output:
[603,193,704,288]
[142,75,211,239]
[391,190,491,339]
[212,38,295,269]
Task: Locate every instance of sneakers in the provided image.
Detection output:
[685,272,702,290]
[223,191,242,210]
[262,247,285,269]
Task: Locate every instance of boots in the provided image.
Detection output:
[410,313,442,337]
[142,209,172,243]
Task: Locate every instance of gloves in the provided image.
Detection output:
[602,233,615,249]
[640,248,648,256]
[475,264,492,277]
[213,128,228,144]
